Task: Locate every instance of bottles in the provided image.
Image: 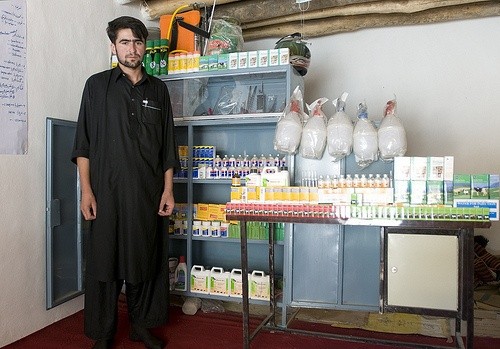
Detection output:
[318,171,393,188]
[229,268,250,298]
[210,267,230,297]
[301,171,317,187]
[192,220,228,238]
[111,52,118,68]
[168,51,200,74]
[142,39,168,77]
[169,256,187,291]
[231,171,241,187]
[190,265,211,294]
[261,167,290,187]
[246,168,261,187]
[168,217,187,235]
[206,154,285,178]
[250,270,270,301]
[228,220,284,241]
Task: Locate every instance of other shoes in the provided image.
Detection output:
[91,339,113,349]
[129,326,163,349]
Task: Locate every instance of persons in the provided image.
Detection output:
[71,16,183,349]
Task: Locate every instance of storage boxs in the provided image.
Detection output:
[393,156,500,221]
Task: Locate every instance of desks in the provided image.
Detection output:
[226,215,492,349]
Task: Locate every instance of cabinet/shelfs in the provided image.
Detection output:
[157,64,305,329]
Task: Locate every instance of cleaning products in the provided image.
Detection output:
[209,267,230,297]
[230,268,251,297]
[379,99,408,161]
[174,256,187,292]
[250,270,270,301]
[327,92,353,163]
[299,97,327,160]
[274,85,302,155]
[189,265,210,295]
[353,101,379,168]
[244,166,291,187]
